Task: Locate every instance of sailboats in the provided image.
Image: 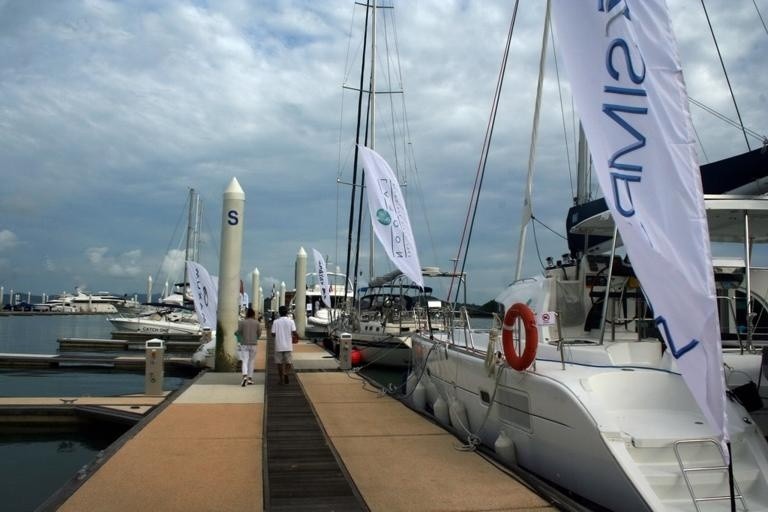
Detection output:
[400,0,768,512]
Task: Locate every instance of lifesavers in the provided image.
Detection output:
[502,303,539,371]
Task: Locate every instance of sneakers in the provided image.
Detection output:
[248,379,254,384]
[240,376,246,386]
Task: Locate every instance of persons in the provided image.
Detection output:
[263,309,268,328]
[271,312,275,324]
[313,296,321,311]
[270,305,297,385]
[236,309,262,386]
